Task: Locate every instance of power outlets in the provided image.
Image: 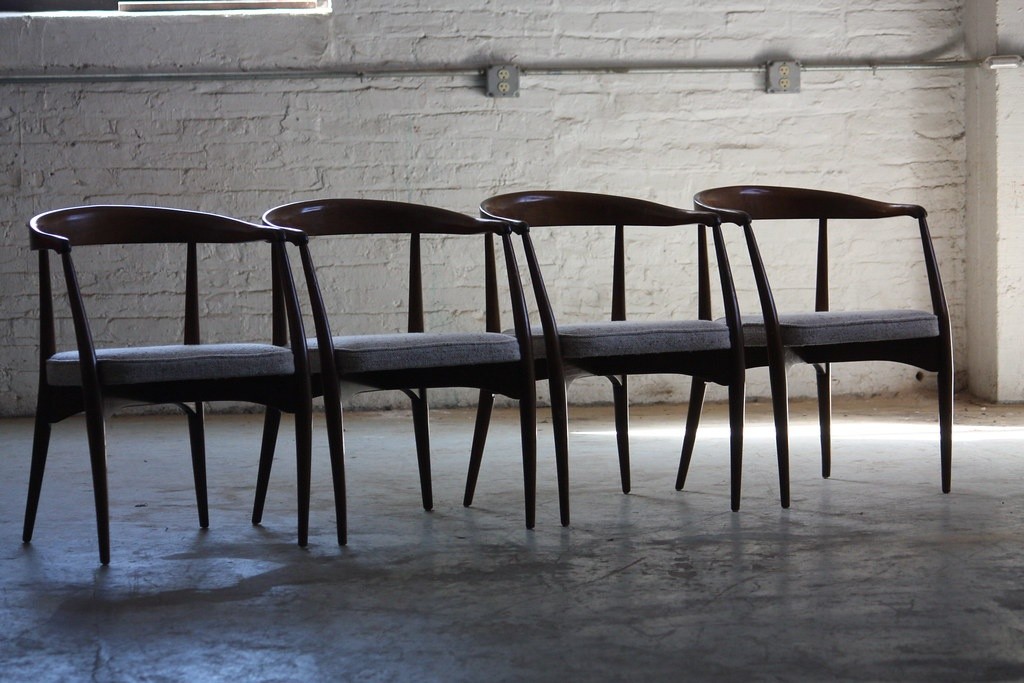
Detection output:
[765,60,801,94]
[482,64,521,98]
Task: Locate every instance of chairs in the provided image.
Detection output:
[676,186,955,508]
[23,204,314,563]
[459,189,746,528]
[252,197,537,547]
